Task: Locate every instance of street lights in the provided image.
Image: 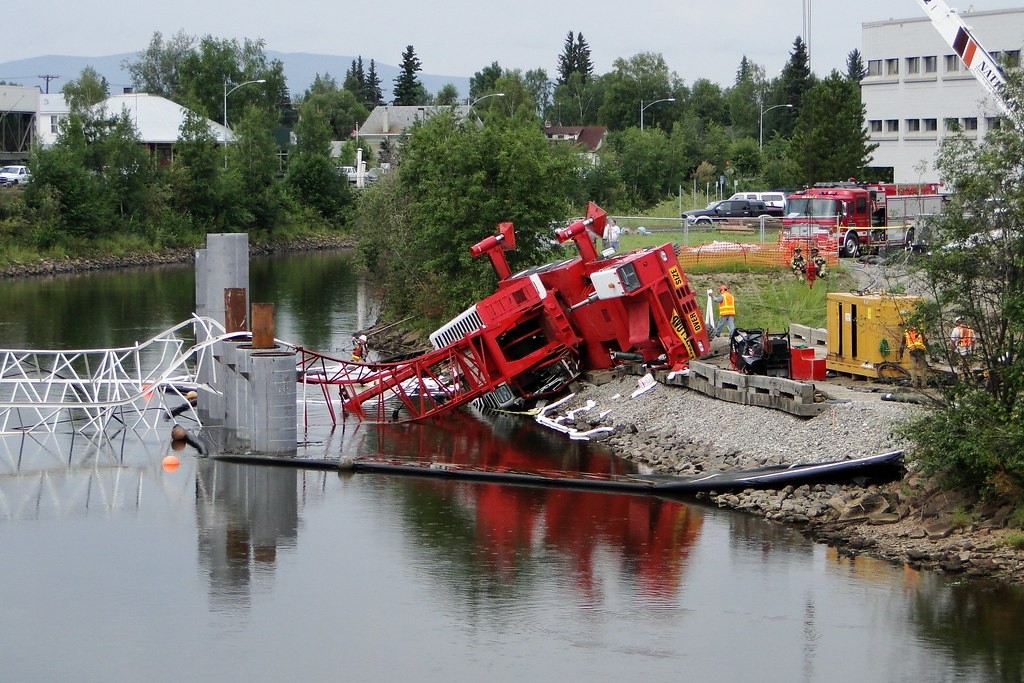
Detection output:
[223,79,268,171]
[417,107,425,124]
[639,98,676,132]
[467,93,505,119]
[759,103,792,153]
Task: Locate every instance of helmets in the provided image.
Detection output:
[955,316,963,324]
[811,246,819,251]
[794,247,802,252]
[359,335,367,342]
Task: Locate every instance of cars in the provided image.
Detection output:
[0,166,36,187]
[368,168,385,183]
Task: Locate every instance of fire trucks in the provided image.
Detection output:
[782,177,949,259]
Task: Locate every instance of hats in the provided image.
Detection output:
[719,285,726,292]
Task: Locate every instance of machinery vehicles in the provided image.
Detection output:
[336,199,719,432]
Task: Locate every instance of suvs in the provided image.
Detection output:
[336,167,358,184]
[706,191,787,214]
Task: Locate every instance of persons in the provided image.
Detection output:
[602,220,620,253]
[809,246,830,281]
[790,246,807,283]
[900,322,932,388]
[949,316,976,369]
[550,224,566,260]
[709,285,736,337]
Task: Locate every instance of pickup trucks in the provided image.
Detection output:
[681,200,785,226]
